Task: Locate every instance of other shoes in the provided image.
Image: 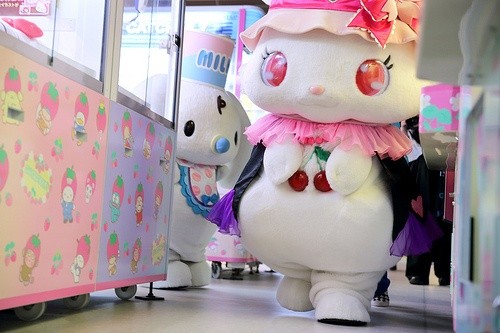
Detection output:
[370,291,392,307]
[409,271,450,286]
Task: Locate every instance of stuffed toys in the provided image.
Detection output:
[145,29,252,289]
[207,0,439,325]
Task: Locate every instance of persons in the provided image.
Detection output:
[373,118,450,306]
